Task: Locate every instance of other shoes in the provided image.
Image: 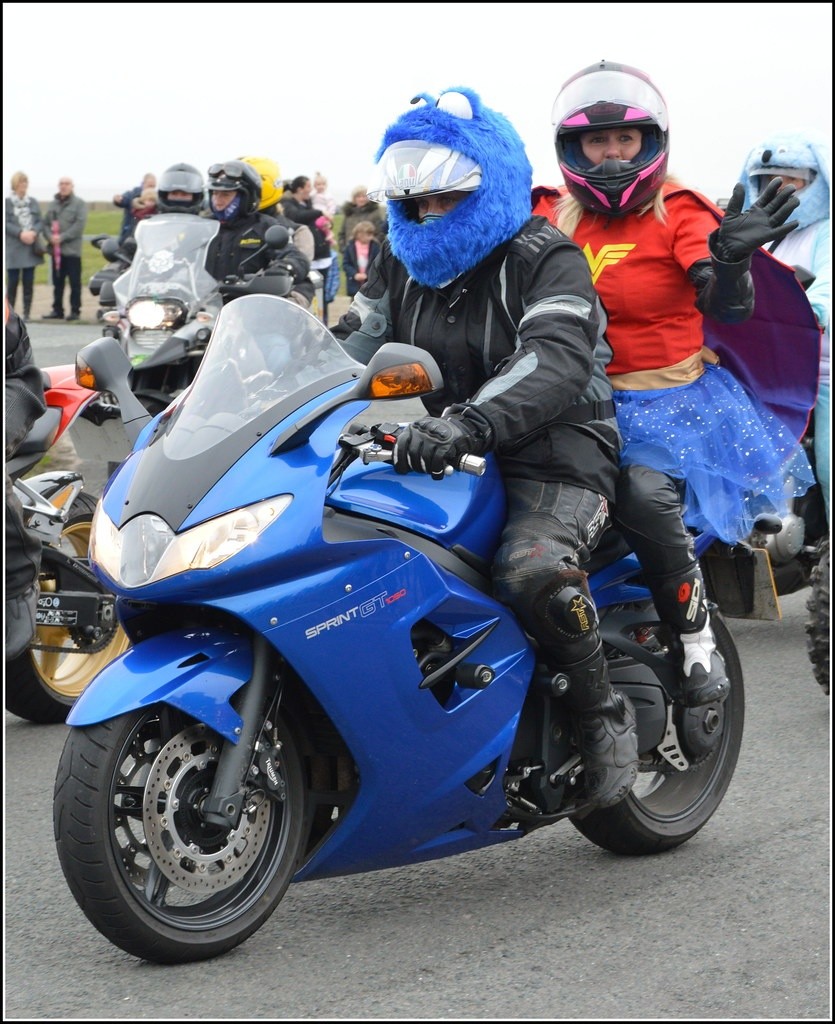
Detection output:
[66,313,80,321]
[6,582,41,663]
[42,310,65,319]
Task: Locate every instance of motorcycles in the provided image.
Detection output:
[5,387,135,723]
[54,296,745,966]
[100,214,290,422]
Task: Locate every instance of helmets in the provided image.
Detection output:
[156,162,206,215]
[552,58,672,218]
[750,165,817,199]
[365,139,482,226]
[202,160,262,226]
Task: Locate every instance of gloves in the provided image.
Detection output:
[715,176,801,264]
[391,412,486,485]
[260,262,299,283]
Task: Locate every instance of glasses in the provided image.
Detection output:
[208,164,258,183]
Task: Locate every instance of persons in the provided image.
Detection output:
[273,89,639,805]
[527,58,817,707]
[110,158,388,330]
[730,143,830,532]
[5,301,45,662]
[4,171,86,323]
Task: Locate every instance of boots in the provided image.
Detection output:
[549,637,640,810]
[643,560,731,707]
